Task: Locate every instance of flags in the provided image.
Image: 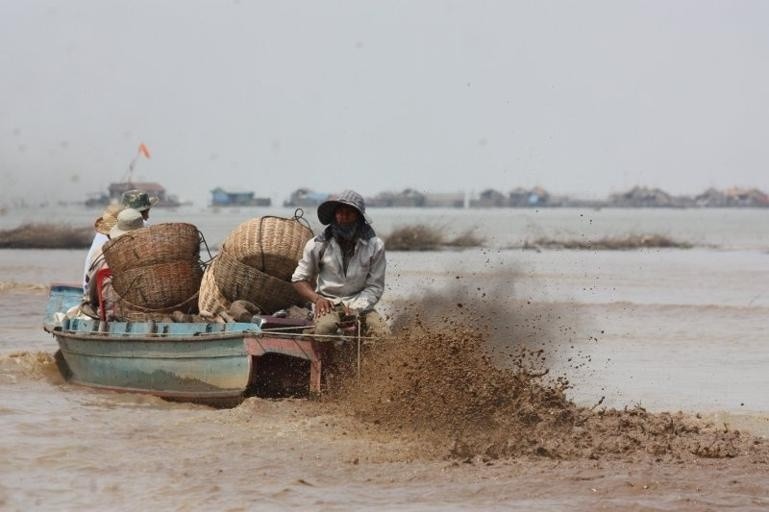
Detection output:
[138,143,150,158]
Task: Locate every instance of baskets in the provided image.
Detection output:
[198,216,316,324]
[102,222,204,323]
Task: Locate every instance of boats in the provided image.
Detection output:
[42,280,375,406]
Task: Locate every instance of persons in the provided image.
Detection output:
[83,190,160,311]
[290,189,392,367]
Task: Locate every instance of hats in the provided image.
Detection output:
[317,190,375,240]
[93,214,116,235]
[104,190,159,217]
[107,208,149,239]
